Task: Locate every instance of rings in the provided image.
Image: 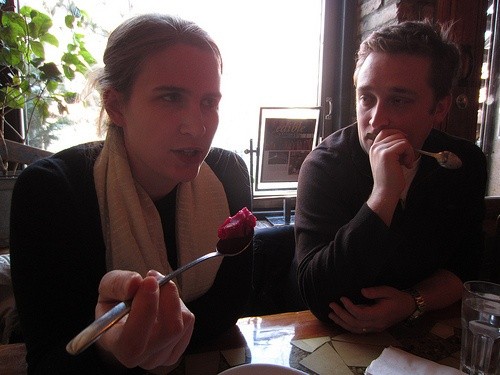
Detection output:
[362,328,367,335]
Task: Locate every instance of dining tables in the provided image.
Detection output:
[0,309,462,375]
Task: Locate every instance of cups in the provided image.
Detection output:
[460,281,500,375]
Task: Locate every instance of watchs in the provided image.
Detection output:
[409,288,426,323]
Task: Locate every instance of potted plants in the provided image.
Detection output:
[0,6,98,248]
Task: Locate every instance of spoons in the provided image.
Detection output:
[64,227,253,355]
[367,131,463,170]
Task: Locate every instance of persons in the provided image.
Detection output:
[8,14,254,375]
[293,19,490,335]
